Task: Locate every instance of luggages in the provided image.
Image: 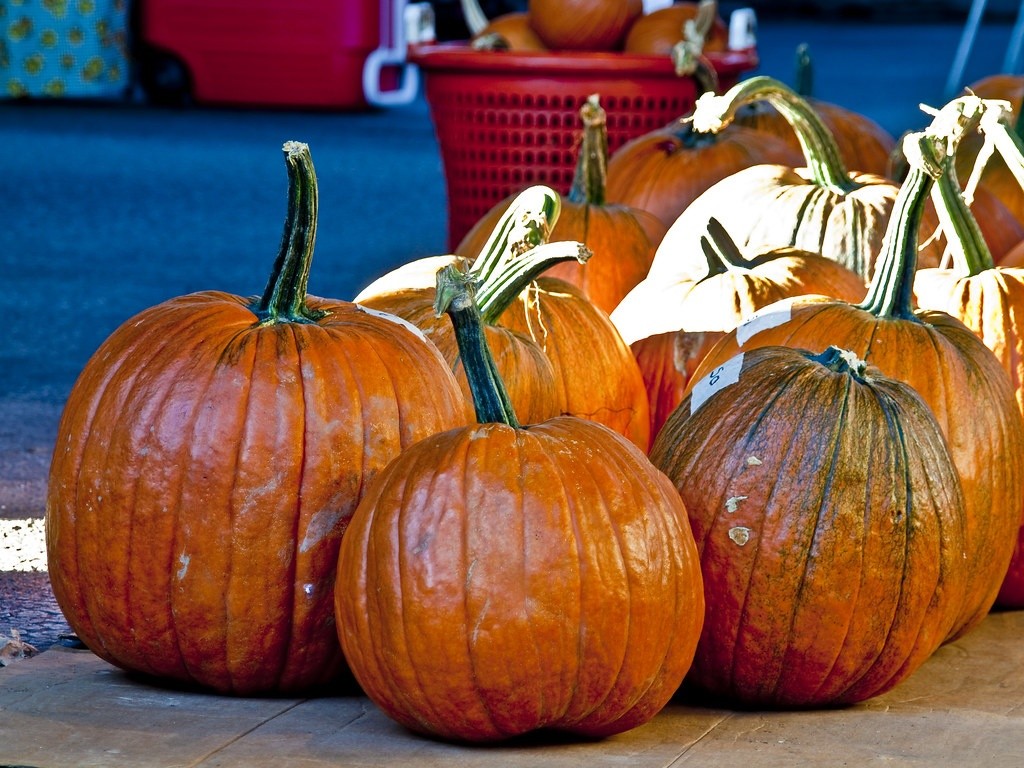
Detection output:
[129,0,403,109]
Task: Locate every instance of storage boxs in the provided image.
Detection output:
[406,37,760,256]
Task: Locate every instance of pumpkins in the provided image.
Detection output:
[45,0,1024,738]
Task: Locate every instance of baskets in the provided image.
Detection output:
[405,44,758,256]
